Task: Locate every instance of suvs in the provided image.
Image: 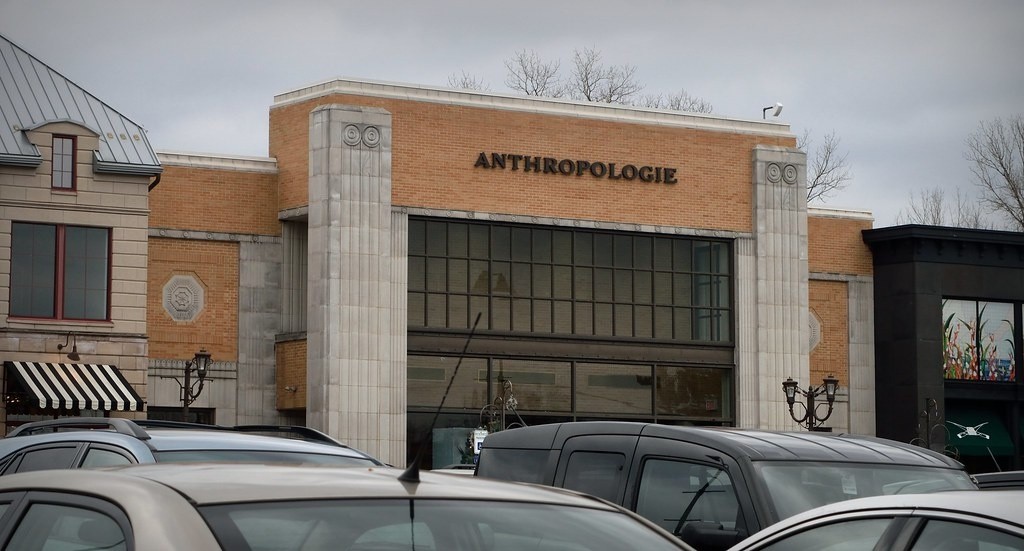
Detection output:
[0,416,392,551]
[475,422,980,551]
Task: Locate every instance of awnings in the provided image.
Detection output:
[5,359,144,412]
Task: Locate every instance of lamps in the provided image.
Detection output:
[57,330,81,361]
[285,386,297,392]
[763,103,783,119]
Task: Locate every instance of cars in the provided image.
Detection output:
[725,491,1024,551]
[0,465,698,551]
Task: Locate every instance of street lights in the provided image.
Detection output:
[159,348,213,422]
